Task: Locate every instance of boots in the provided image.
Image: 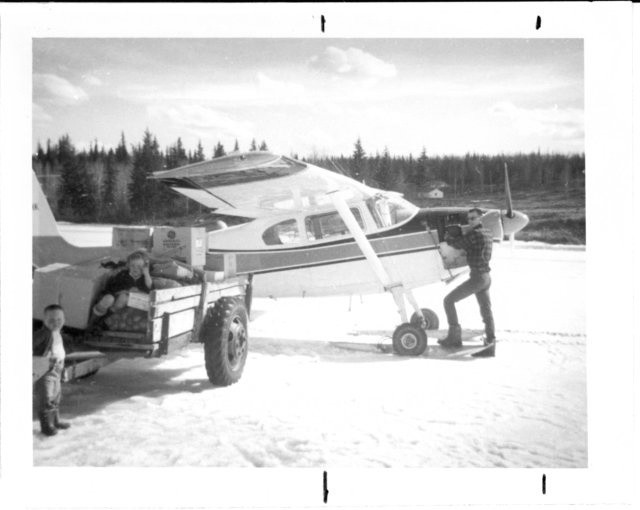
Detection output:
[471,338,495,357]
[55,409,70,429]
[95,309,115,331]
[89,306,107,332]
[39,409,58,434]
[438,325,462,347]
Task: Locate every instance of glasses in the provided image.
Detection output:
[465,216,480,220]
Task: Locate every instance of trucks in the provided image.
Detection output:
[62,263,250,387]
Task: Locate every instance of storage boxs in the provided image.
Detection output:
[111,226,151,250]
[153,226,207,267]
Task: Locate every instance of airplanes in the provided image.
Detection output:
[32,150,530,357]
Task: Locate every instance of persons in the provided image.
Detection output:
[88,250,156,330]
[32,304,70,436]
[437,208,496,357]
